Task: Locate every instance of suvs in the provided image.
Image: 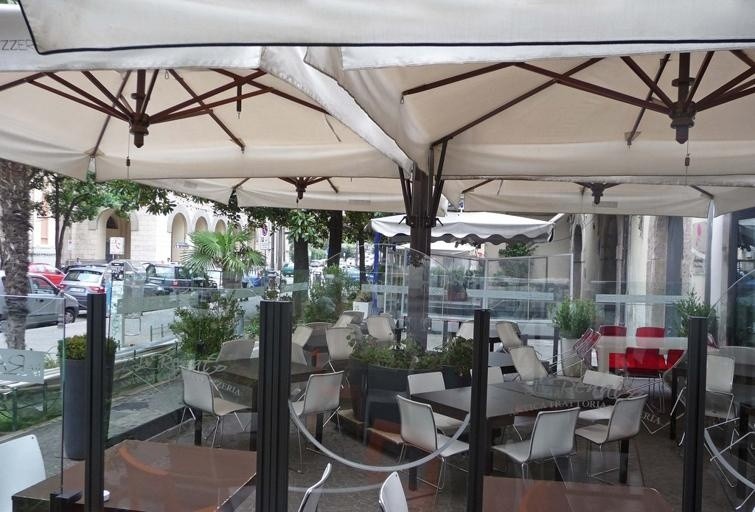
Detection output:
[26,261,68,291]
[63,260,294,315]
[0,272,80,327]
[309,259,328,277]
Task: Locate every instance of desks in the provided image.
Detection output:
[360,327,407,345]
[489,331,528,352]
[409,377,630,491]
[671,352,754,440]
[596,336,720,373]
[482,475,676,512]
[488,351,549,375]
[11,439,257,512]
[303,335,328,367]
[195,358,327,451]
[736,399,755,499]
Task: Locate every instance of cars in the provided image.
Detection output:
[342,267,374,284]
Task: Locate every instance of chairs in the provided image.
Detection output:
[452,321,474,342]
[396,394,469,506]
[509,346,549,381]
[579,370,624,421]
[573,328,594,352]
[636,327,666,371]
[470,367,504,384]
[0,434,47,511]
[577,331,601,371]
[670,354,737,451]
[176,366,252,447]
[292,370,344,470]
[379,472,408,512]
[291,325,314,366]
[625,347,662,415]
[575,394,649,485]
[216,340,255,362]
[596,325,627,369]
[496,322,524,351]
[491,407,580,482]
[719,345,754,365]
[343,311,364,324]
[363,364,413,447]
[333,316,353,329]
[325,327,356,360]
[305,322,332,335]
[667,350,684,369]
[407,372,471,438]
[297,463,333,512]
[368,317,394,340]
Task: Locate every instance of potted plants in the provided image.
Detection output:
[346,331,378,421]
[56,335,119,460]
[547,294,603,377]
